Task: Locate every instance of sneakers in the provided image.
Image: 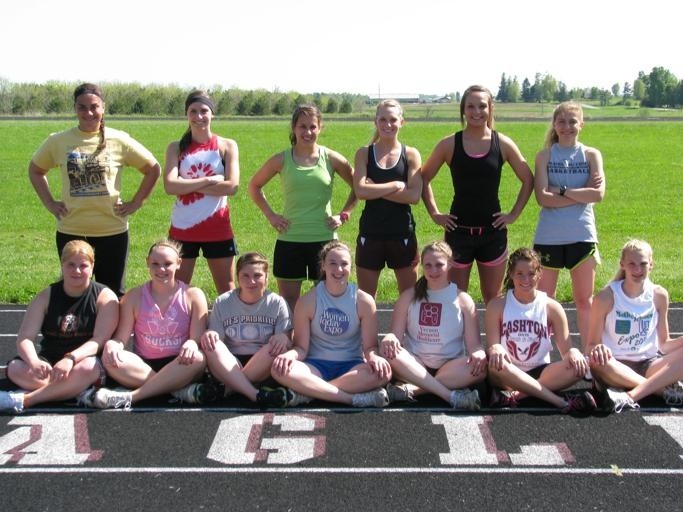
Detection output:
[352,386,390,408]
[169,382,198,405]
[386,382,413,404]
[63,384,96,409]
[558,390,598,414]
[449,387,482,411]
[256,385,288,409]
[286,387,315,408]
[488,387,521,408]
[91,385,132,409]
[602,388,635,414]
[0,390,30,415]
[195,382,231,406]
[663,382,683,406]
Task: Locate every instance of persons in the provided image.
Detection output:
[270,242,392,407]
[0,241,119,413]
[586,239,683,414]
[92,240,207,408]
[380,241,488,410]
[424,86,533,306]
[535,100,606,355]
[248,105,358,312]
[200,253,293,408]
[29,84,160,302]
[165,91,239,294]
[486,248,597,417]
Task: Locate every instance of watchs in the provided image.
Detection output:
[340,211,349,222]
[560,185,567,195]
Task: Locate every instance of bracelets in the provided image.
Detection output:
[64,353,77,366]
[353,99,422,300]
